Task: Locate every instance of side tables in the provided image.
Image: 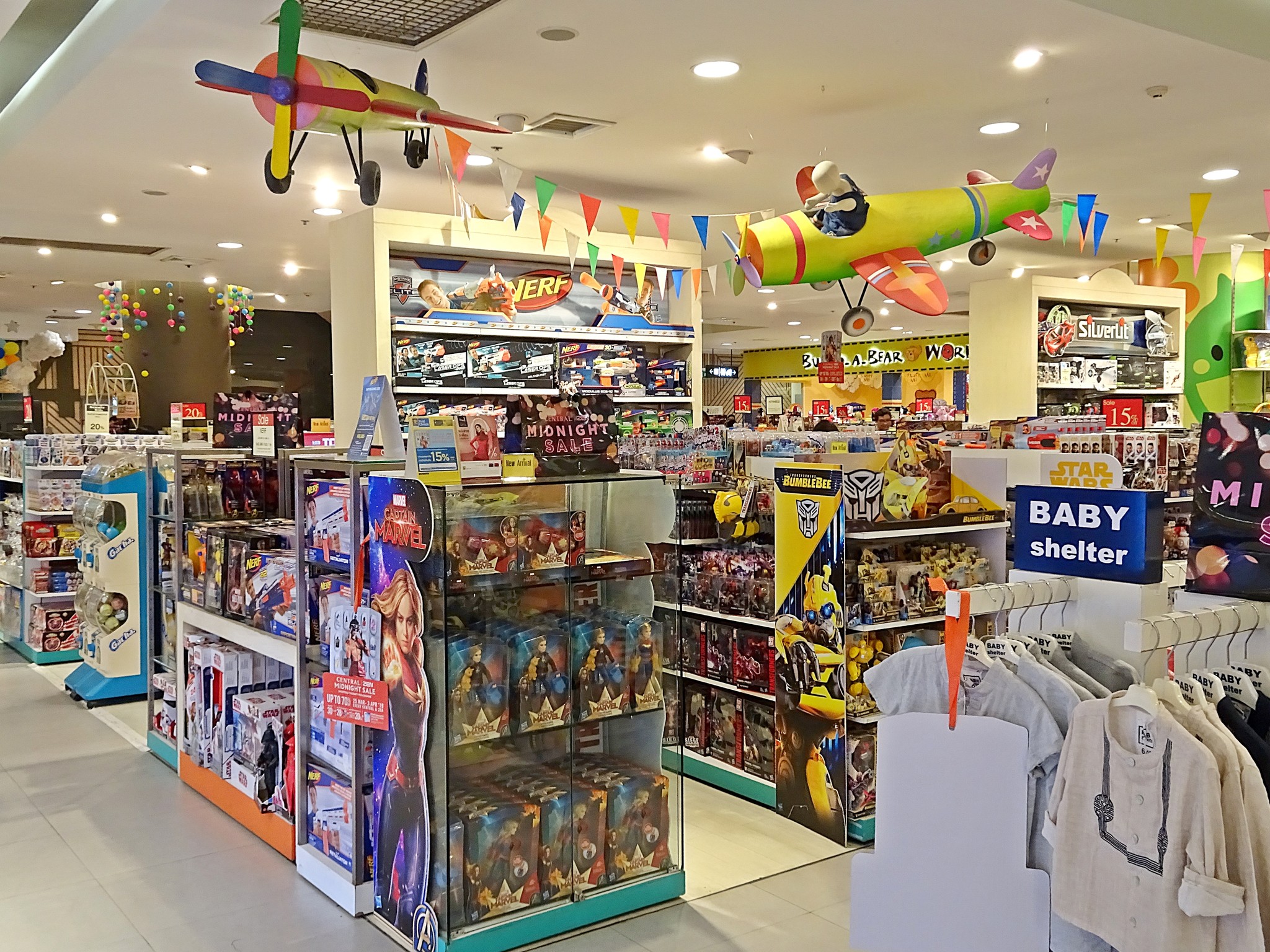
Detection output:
[59,450,174,710]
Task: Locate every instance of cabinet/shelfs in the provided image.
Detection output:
[1229,276,1270,412]
[968,267,1186,427]
[654,449,1011,843]
[1,434,177,664]
[328,209,702,445]
[144,449,684,951]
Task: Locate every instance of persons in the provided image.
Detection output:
[724,414,736,427]
[635,278,655,323]
[434,787,654,920]
[456,624,662,722]
[804,160,869,236]
[398,343,499,374]
[659,572,773,765]
[420,436,428,448]
[1061,441,1168,491]
[243,494,371,848]
[397,406,408,422]
[417,279,517,321]
[1038,361,1079,383]
[875,407,892,430]
[812,419,840,432]
[446,512,585,577]
[256,729,279,800]
[470,424,489,461]
[852,569,935,774]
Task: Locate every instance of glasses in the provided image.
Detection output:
[878,418,890,424]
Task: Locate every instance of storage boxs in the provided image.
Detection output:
[1,335,1199,932]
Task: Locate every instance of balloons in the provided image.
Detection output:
[0,338,20,370]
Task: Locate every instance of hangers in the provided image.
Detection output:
[883,577,1270,770]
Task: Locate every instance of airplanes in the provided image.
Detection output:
[195,0,514,206]
[722,146,1058,337]
